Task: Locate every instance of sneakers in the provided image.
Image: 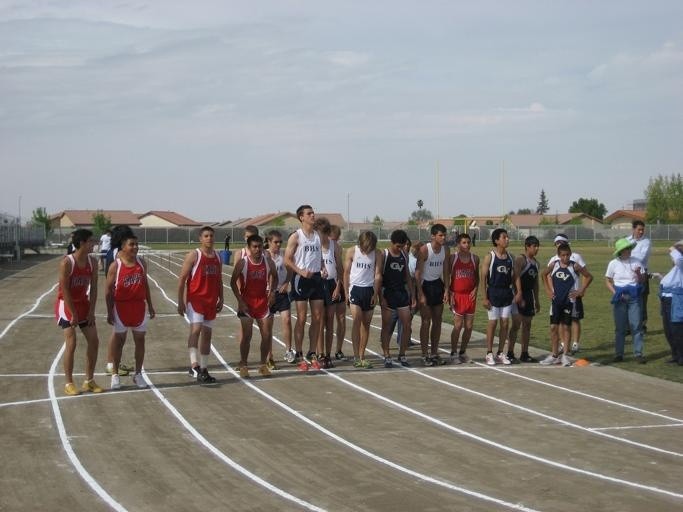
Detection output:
[539,342,578,366]
[284,350,348,370]
[422,352,471,366]
[64,383,80,395]
[258,366,271,376]
[486,351,533,365]
[106,363,133,376]
[240,364,249,378]
[353,357,372,368]
[384,356,393,368]
[398,354,408,366]
[267,359,277,370]
[111,374,121,390]
[134,373,147,388]
[83,381,102,393]
[189,366,215,384]
[636,356,646,363]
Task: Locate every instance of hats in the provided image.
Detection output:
[614,238,634,257]
[554,236,568,244]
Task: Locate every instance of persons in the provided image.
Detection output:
[605,238,646,363]
[648,240,683,366]
[539,243,593,366]
[506,236,540,364]
[481,229,516,365]
[547,234,587,355]
[55,225,155,396]
[224,234,231,251]
[229,204,479,380]
[177,227,223,384]
[624,221,651,337]
